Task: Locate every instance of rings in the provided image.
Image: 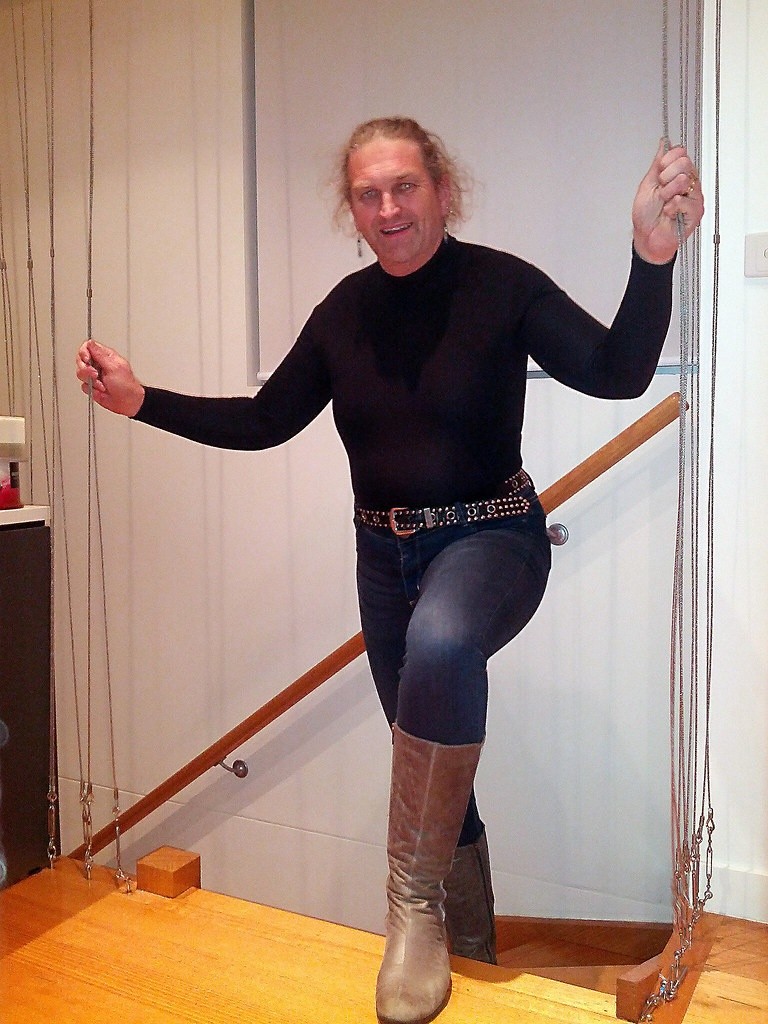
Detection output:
[682,179,695,197]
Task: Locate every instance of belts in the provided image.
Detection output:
[354,467,533,535]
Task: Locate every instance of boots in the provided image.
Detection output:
[446,831,498,965]
[374,720,488,1022]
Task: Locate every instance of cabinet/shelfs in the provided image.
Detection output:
[0,501,61,889]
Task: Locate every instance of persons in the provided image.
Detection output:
[77,115,703,1024]
